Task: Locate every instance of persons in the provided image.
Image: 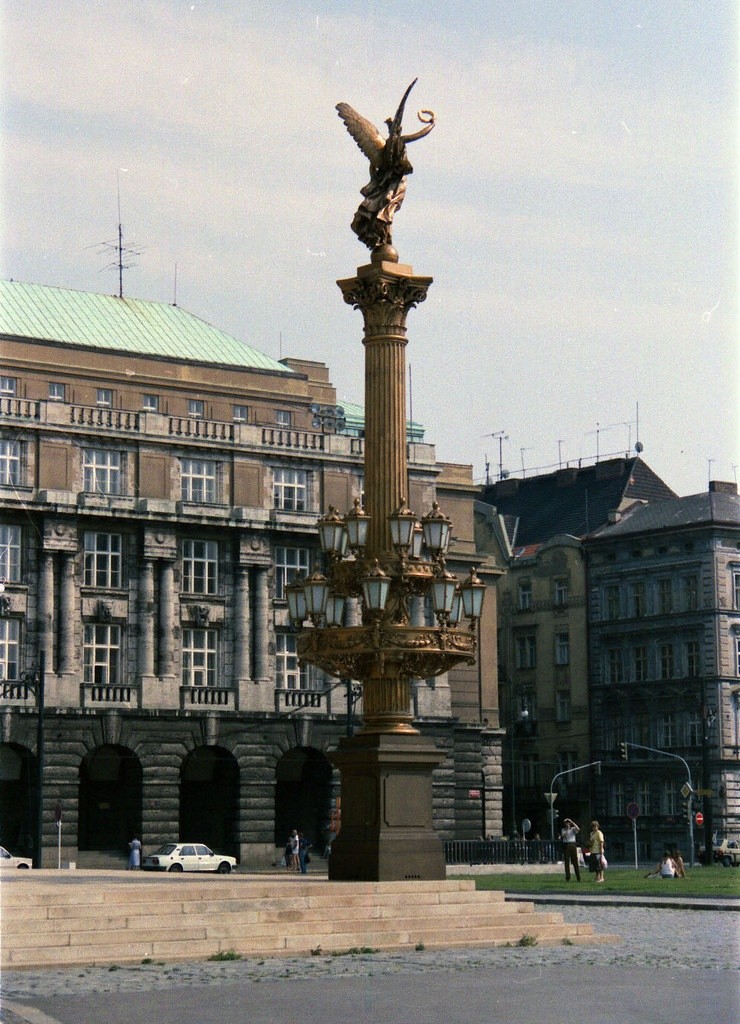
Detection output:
[531,834,545,862]
[644,850,686,878]
[587,821,605,883]
[128,835,141,871]
[465,833,494,865]
[351,117,436,244]
[562,818,581,882]
[555,835,561,859]
[576,842,589,867]
[284,830,312,874]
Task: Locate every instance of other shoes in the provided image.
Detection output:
[596,879,604,883]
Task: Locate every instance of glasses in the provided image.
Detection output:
[591,825,598,828]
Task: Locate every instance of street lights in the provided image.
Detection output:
[284,76,487,879]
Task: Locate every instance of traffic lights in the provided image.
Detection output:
[616,741,628,762]
[594,761,601,776]
[513,695,528,723]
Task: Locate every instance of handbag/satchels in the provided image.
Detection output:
[596,853,608,869]
[281,855,287,866]
[305,851,311,864]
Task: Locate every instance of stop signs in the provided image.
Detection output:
[695,812,703,825]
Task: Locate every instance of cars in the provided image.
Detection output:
[697,838,740,866]
[138,841,238,875]
[0,845,33,870]
